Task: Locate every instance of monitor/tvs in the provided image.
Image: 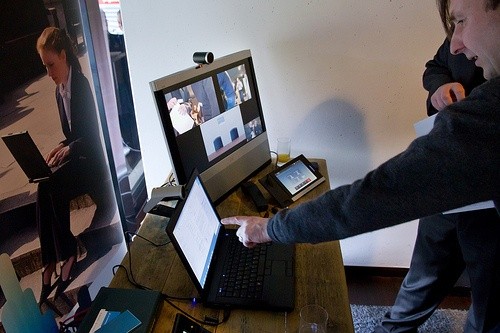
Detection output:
[148,49,272,208]
[270,154,327,202]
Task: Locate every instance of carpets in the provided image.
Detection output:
[348,303,471,333]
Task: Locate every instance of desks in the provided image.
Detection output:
[108,157,354,333]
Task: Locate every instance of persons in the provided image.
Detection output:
[222,0,500,333]
[217,72,235,110]
[164,87,190,135]
[249,120,262,139]
[236,78,245,102]
[191,75,219,122]
[243,74,252,99]
[36,26,109,306]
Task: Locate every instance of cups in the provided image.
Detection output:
[298,304,328,333]
[277,136,291,168]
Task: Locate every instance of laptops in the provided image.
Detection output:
[0,131,73,183]
[165,167,297,313]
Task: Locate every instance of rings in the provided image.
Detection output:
[239,237,242,239]
